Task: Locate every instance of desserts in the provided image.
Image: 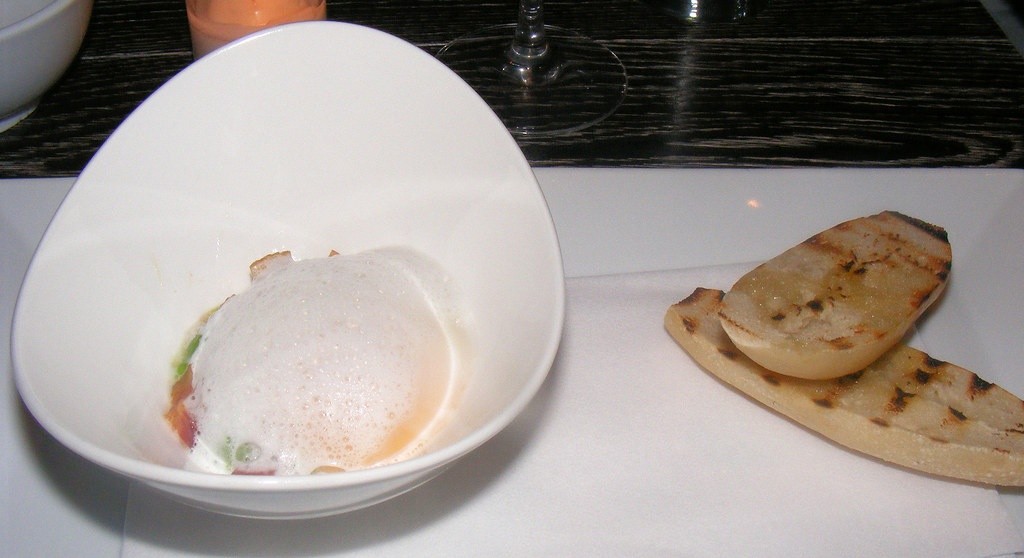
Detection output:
[165,246,449,476]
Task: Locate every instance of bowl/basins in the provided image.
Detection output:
[0,0,93,131]
[11,21,567,521]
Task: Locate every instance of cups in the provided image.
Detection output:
[187,0,327,62]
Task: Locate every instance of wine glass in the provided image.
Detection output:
[435,0,628,134]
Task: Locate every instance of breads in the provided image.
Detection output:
[718,211,952,379]
[663,286,1024,487]
